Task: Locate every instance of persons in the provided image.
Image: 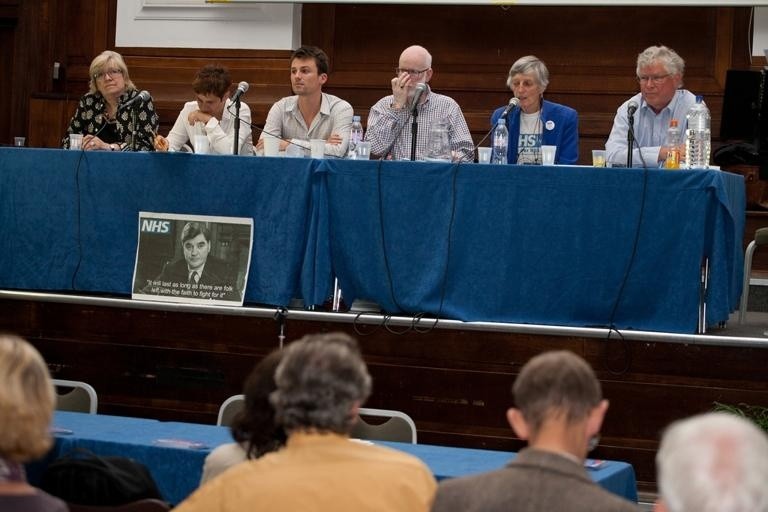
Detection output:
[154,67,253,156]
[0,334,69,511]
[61,50,159,152]
[168,333,437,511]
[364,45,475,163]
[200,350,287,488]
[141,222,241,301]
[604,46,709,168]
[490,56,579,165]
[434,350,650,512]
[654,414,768,511]
[256,47,354,158]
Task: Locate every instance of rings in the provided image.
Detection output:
[400,86,404,89]
[90,140,94,146]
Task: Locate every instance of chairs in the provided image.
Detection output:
[740,225,767,338]
[51,378,99,416]
[358,407,417,444]
[217,395,246,427]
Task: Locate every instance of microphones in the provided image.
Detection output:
[227,81,250,110]
[409,83,426,113]
[119,90,151,110]
[626,100,639,116]
[503,97,519,115]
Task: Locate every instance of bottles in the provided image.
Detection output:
[664,119,682,168]
[491,118,509,166]
[686,95,711,167]
[347,115,363,159]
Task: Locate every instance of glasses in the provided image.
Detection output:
[635,72,670,85]
[394,68,429,79]
[94,68,121,79]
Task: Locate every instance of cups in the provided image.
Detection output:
[14,136,25,147]
[194,135,209,152]
[263,137,279,156]
[592,150,606,167]
[358,143,371,160]
[69,134,83,149]
[311,139,324,158]
[541,145,556,166]
[478,146,493,164]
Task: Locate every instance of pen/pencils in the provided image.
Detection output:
[151,130,168,153]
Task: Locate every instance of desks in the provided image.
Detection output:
[25,410,638,512]
[1,148,744,335]
